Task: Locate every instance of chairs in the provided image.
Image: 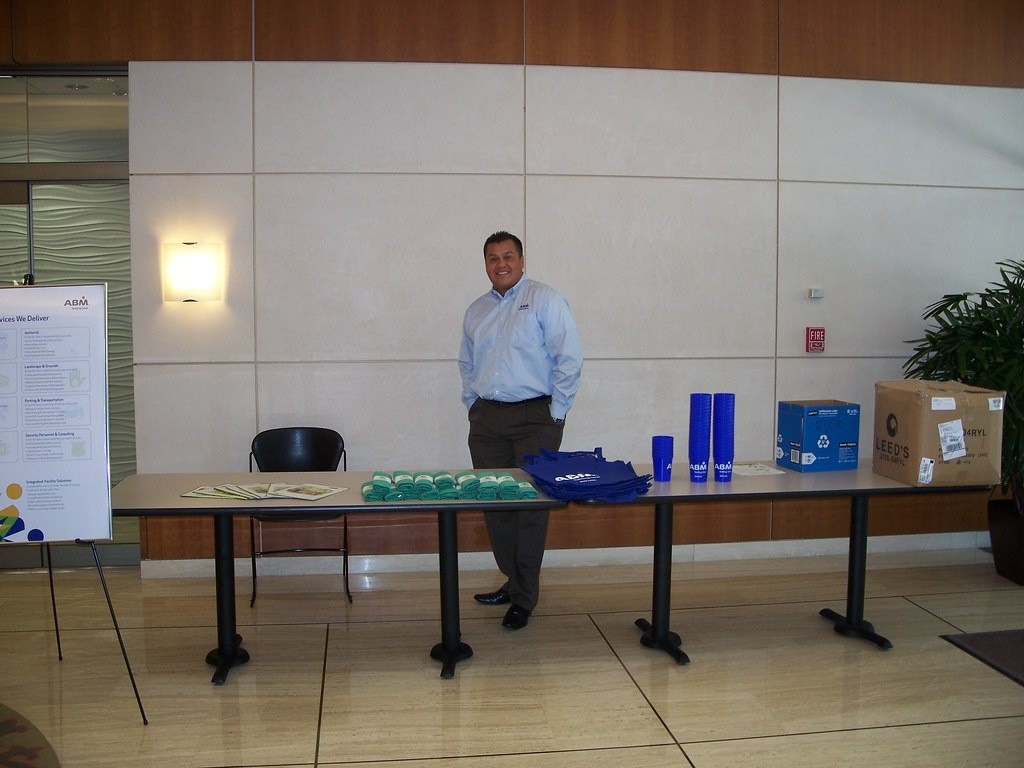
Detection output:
[251,427,353,608]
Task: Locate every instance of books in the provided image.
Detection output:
[180,482,350,500]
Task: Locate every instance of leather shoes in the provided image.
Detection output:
[474,586,512,605]
[501,603,532,629]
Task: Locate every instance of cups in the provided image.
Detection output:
[651,435,674,482]
[688,393,736,483]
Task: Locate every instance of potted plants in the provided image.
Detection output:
[902,258,1024,586]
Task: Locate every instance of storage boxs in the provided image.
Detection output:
[872,379,1007,486]
[778,399,861,472]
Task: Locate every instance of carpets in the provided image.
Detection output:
[938,629,1024,688]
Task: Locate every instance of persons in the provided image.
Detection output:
[457,231,583,629]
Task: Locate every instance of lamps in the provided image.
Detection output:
[161,239,224,303]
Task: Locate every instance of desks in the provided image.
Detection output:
[112,460,996,684]
